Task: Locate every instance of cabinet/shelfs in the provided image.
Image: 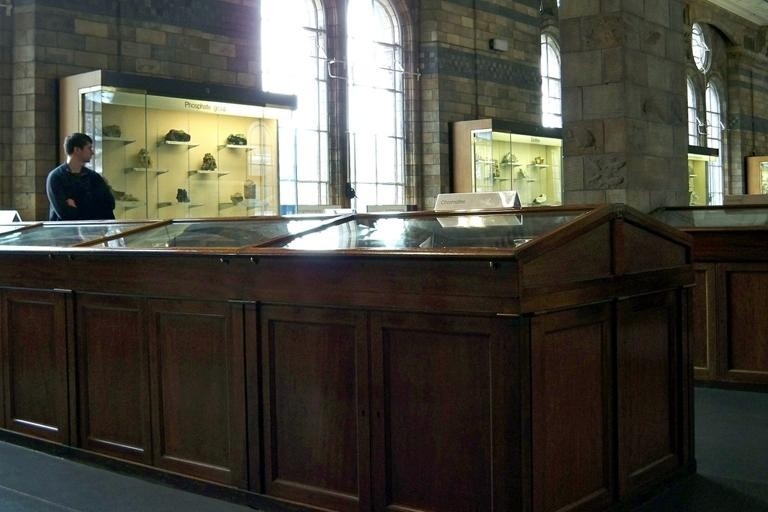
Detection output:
[746,155,767,194]
[520,201,696,512]
[684,145,715,205]
[238,246,521,511]
[58,68,284,222]
[63,248,238,506]
[687,228,767,393]
[449,118,564,203]
[0,250,63,460]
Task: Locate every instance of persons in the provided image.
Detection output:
[42,133,118,221]
